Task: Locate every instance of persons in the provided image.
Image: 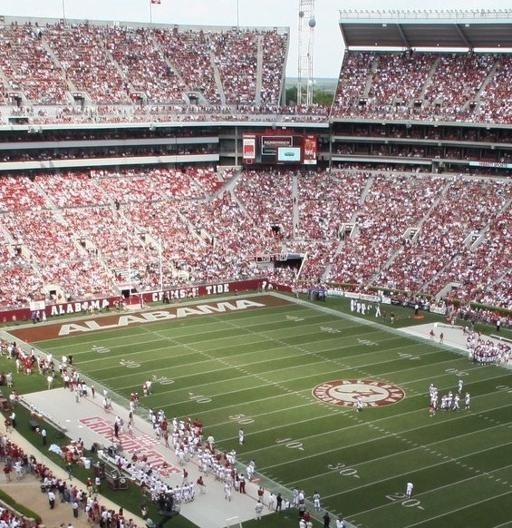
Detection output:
[404,479,413,499]
[0,15,329,323]
[427,376,472,419]
[329,52,512,367]
[354,400,363,413]
[0,338,346,528]
